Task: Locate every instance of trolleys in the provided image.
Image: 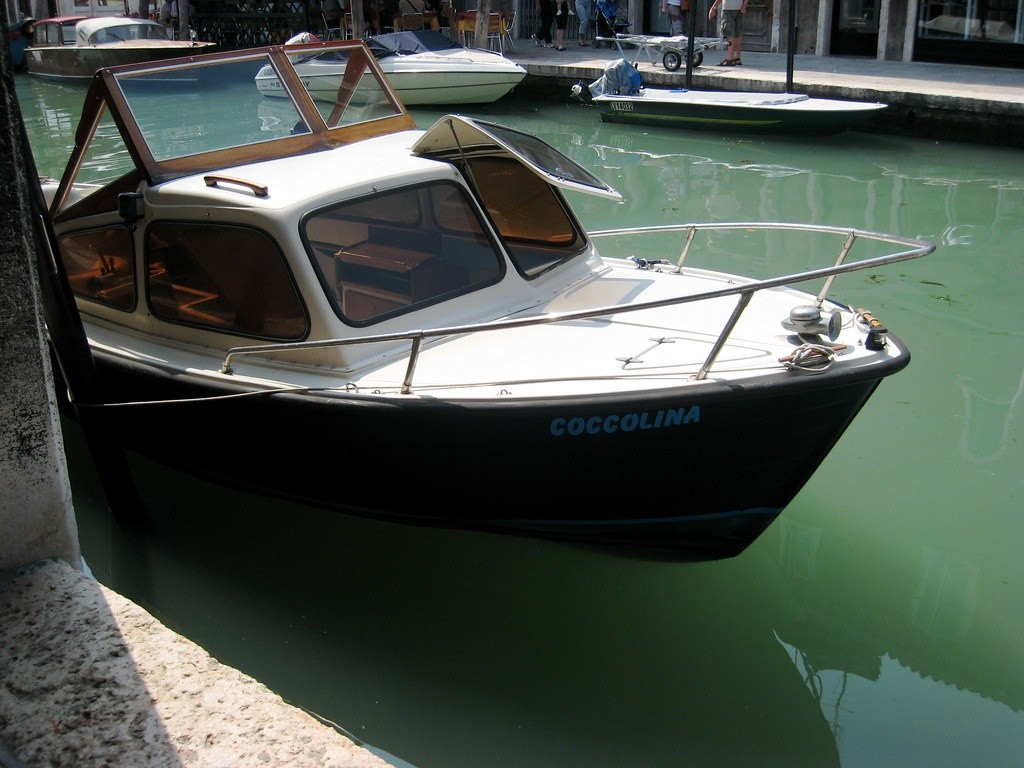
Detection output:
[594,33,729,71]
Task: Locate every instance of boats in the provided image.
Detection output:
[254,31,530,106]
[38,42,941,564]
[1,0,39,73]
[26,15,219,85]
[571,0,890,129]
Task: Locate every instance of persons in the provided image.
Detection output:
[575,0,592,46]
[399,0,431,31]
[556,0,575,16]
[709,0,749,66]
[160,0,323,46]
[323,0,350,40]
[551,0,568,51]
[661,0,690,37]
[530,0,556,48]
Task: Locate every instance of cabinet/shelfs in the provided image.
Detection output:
[335,242,441,321]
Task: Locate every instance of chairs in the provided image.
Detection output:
[321,8,517,56]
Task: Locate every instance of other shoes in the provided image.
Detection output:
[568,10,574,14]
[577,41,588,46]
[555,46,567,51]
[556,10,562,15]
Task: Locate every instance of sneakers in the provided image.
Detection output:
[543,43,555,47]
[531,34,542,47]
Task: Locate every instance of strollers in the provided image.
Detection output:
[591,1,637,50]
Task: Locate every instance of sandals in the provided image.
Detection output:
[735,57,742,65]
[717,59,737,66]
